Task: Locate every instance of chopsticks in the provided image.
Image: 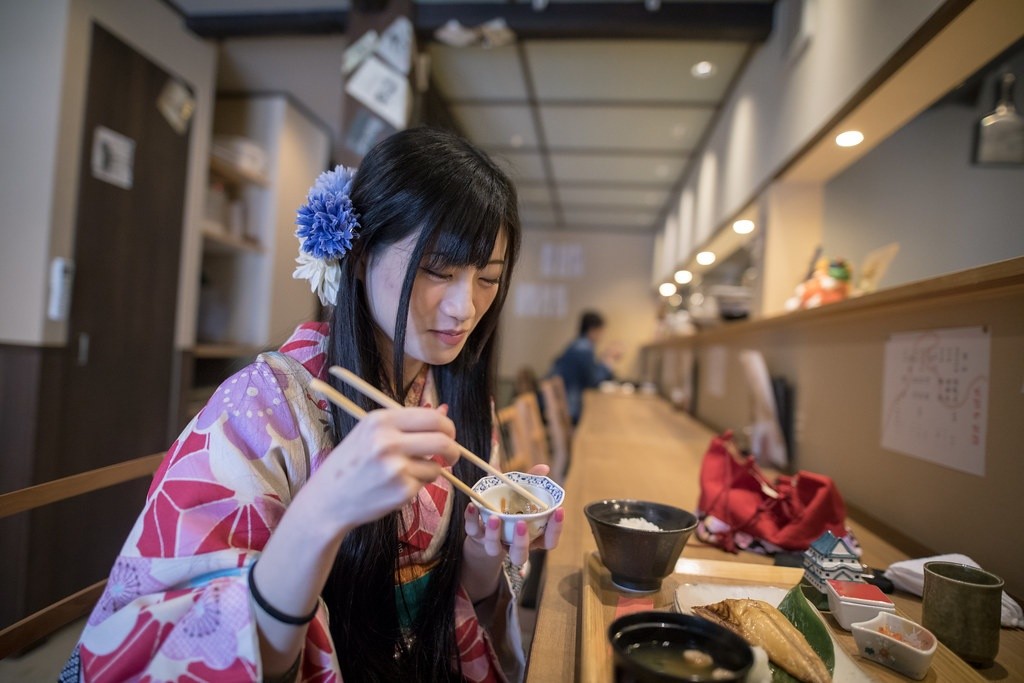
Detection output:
[309,364,549,514]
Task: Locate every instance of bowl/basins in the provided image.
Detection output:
[826,580,895,631]
[583,500,699,593]
[470,473,566,547]
[607,611,753,683]
[850,611,938,679]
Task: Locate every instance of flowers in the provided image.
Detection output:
[292,166,359,308]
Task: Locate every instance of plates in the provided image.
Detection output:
[674,584,873,683]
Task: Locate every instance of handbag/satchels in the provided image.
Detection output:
[691,429,848,557]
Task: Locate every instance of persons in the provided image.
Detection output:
[59,126,563,683]
[538,310,613,426]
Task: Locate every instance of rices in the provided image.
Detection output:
[614,517,664,531]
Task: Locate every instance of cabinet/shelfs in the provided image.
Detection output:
[181,142,270,416]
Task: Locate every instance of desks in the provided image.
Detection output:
[525,373,1024,682]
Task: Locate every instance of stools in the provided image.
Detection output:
[0,377,570,683]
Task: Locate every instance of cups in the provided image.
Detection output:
[921,560,1004,668]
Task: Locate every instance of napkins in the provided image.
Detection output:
[884,552,1024,630]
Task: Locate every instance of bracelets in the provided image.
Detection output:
[248,559,320,625]
[474,595,493,607]
